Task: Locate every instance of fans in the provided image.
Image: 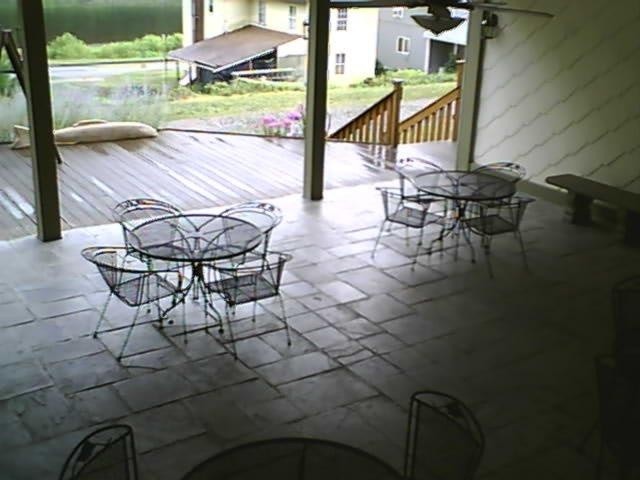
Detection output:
[325,0,557,36]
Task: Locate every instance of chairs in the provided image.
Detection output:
[57,156,640,479]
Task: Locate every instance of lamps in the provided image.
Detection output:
[481,8,499,40]
